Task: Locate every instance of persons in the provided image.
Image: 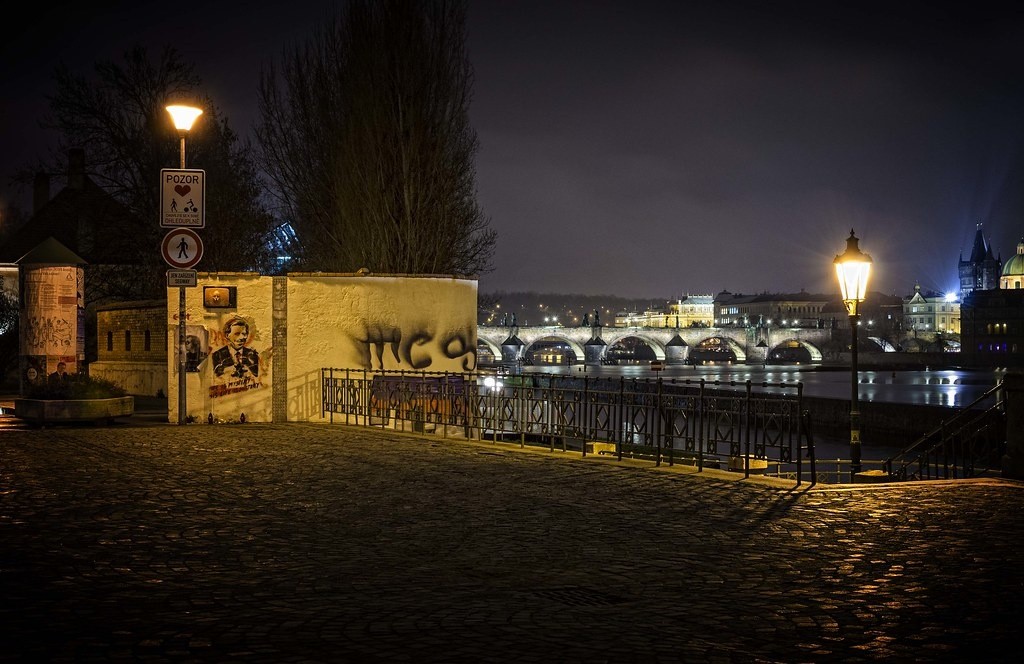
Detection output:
[49,363,67,383]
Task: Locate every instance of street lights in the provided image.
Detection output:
[832,225,874,484]
[166,103,203,170]
